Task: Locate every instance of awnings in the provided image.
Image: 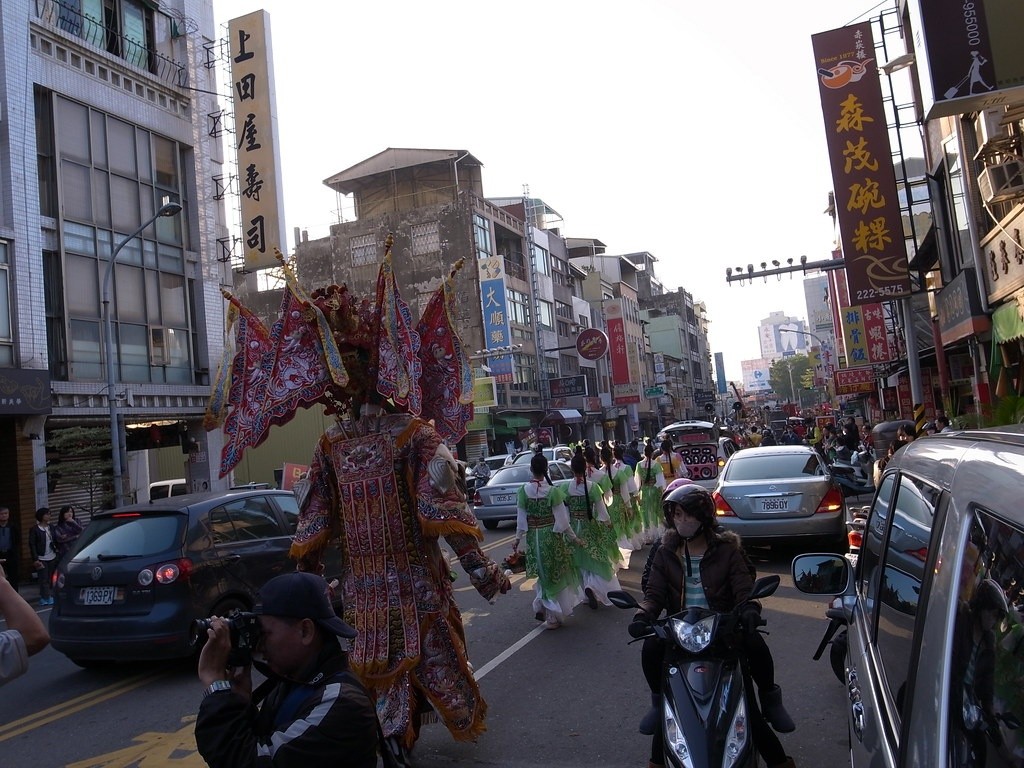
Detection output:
[496,416,531,429]
[548,408,582,425]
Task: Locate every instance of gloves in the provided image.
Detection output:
[628,613,651,638]
[741,601,762,633]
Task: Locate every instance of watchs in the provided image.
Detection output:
[202,680,234,699]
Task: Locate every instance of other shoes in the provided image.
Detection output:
[585,585,598,609]
[39,599,48,605]
[535,611,545,622]
[545,620,562,630]
[48,597,55,605]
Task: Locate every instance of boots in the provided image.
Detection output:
[639,694,663,735]
[758,684,796,733]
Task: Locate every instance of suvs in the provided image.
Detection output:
[790,424,1024,768]
[510,444,575,467]
[661,420,738,492]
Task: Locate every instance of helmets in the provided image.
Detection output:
[663,484,716,528]
[970,578,1009,622]
[661,478,695,500]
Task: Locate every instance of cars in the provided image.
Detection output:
[149,479,187,500]
[712,444,850,552]
[47,490,345,675]
[473,461,574,530]
[484,454,512,478]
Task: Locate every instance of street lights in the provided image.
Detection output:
[104,202,183,508]
[779,329,830,382]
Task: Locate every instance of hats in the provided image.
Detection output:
[252,573,359,639]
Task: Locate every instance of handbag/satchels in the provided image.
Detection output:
[49,541,58,553]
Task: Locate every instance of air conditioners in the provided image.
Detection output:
[974,104,1008,155]
[977,158,1024,203]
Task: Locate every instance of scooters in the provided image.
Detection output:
[606,575,781,768]
[472,474,487,503]
[827,445,875,501]
[845,505,871,569]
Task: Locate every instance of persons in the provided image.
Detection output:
[0,506,20,592]
[628,478,796,768]
[195,571,385,768]
[289,368,510,768]
[879,416,953,473]
[510,440,690,629]
[896,543,1024,768]
[712,400,879,467]
[0,562,52,686]
[472,457,490,489]
[28,505,86,607]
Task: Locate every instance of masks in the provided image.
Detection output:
[673,518,701,537]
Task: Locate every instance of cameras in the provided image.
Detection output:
[190,612,264,666]
